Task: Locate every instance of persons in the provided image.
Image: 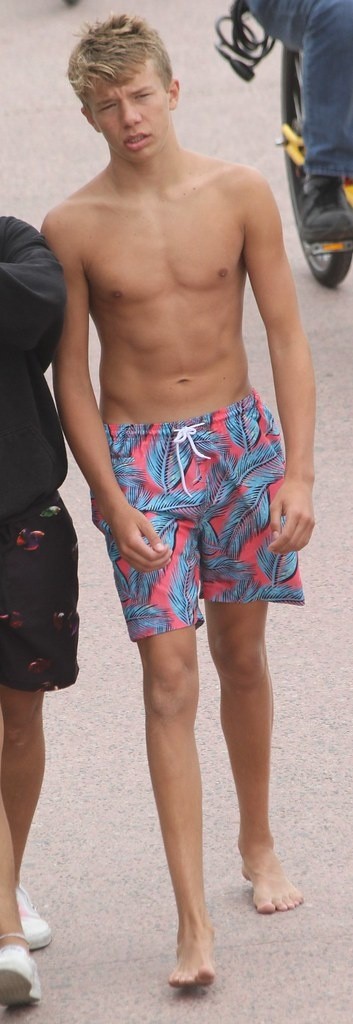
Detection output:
[40,13,315,986]
[245,0,353,244]
[0,216,80,1006]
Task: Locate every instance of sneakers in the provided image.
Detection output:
[0,946,42,1005]
[296,175,352,240]
[16,884,52,949]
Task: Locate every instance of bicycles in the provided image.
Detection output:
[213,0,352,292]
